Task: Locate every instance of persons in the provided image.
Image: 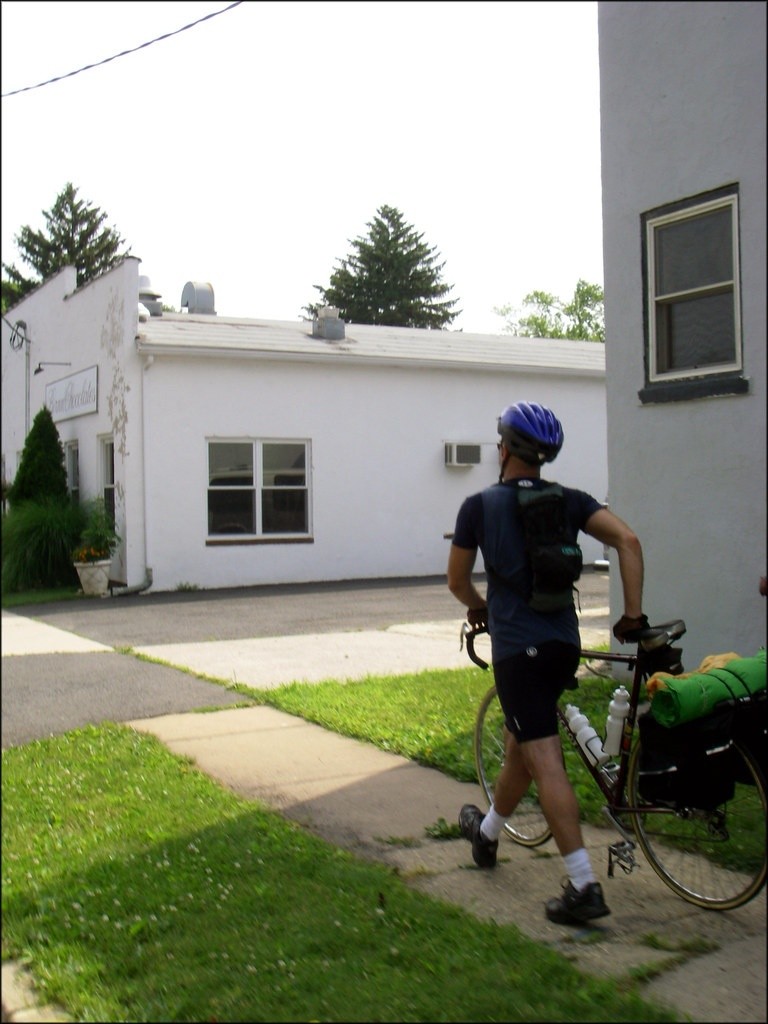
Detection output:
[449,402,651,927]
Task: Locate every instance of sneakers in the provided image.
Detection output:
[544,877,611,924]
[458,803,499,868]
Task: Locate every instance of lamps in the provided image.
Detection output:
[35,362,70,375]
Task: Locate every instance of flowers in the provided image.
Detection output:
[70,494,123,562]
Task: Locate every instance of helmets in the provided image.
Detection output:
[496,399,564,462]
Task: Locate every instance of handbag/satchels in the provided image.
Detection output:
[633,688,768,810]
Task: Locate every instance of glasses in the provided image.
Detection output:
[494,441,501,449]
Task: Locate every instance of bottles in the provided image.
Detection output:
[564,703,609,768]
[602,685,631,758]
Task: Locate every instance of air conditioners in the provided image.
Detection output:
[445,441,481,465]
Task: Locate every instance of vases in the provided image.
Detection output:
[73,559,111,595]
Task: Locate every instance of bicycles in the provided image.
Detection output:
[458,607,768,913]
